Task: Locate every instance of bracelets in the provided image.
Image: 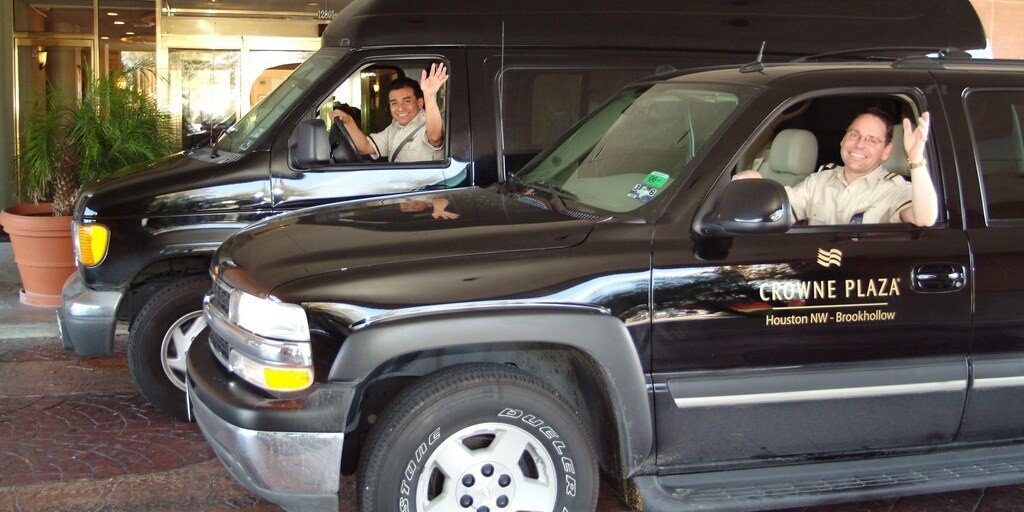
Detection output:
[909,157,926,169]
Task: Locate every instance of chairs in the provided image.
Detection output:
[882,123,928,175]
[747,128,818,189]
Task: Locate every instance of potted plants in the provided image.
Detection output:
[0,52,180,307]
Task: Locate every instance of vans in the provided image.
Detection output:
[56,0,1024,420]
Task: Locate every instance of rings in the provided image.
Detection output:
[921,138,927,141]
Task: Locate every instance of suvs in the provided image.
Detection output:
[185,48,1024,512]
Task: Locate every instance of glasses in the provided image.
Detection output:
[847,131,890,146]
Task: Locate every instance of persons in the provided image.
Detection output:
[732,107,938,227]
[328,62,449,162]
[365,193,460,220]
